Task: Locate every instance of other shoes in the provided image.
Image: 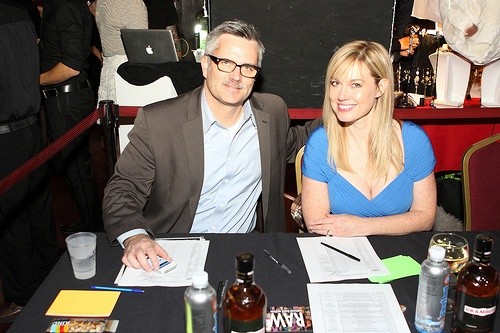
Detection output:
[0,302,25,325]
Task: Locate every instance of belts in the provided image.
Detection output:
[0,115,37,135]
[41,80,91,101]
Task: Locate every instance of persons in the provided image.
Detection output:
[391,35,419,61]
[104,22,323,272]
[92,0,181,126]
[301,41,437,238]
[0,0,103,325]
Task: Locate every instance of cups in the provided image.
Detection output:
[65,231,97,280]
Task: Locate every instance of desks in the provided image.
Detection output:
[7,230,500,333]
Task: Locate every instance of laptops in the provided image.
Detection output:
[119,26,179,63]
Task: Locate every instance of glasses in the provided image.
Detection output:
[207,54,262,79]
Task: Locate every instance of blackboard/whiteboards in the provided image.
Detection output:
[208,0,397,110]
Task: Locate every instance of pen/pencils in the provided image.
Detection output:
[90,285,145,293]
[217,279,228,311]
[320,242,360,262]
[263,249,292,274]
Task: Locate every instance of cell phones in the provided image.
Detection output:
[144,253,177,273]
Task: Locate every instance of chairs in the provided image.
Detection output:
[462,133,500,231]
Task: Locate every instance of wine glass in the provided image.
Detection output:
[426,235,469,314]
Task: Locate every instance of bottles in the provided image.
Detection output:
[223,252,267,333]
[413,245,451,333]
[183,272,218,333]
[453,234,500,333]
